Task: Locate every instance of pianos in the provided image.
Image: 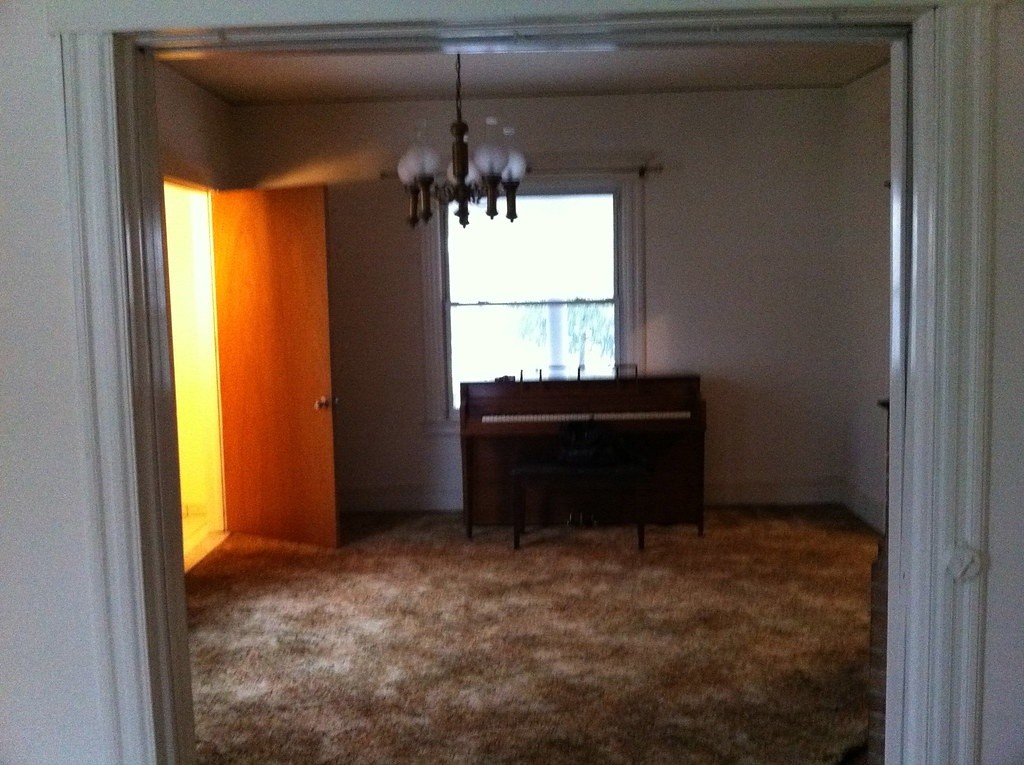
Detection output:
[459,376,707,539]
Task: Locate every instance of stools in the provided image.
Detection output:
[514,447,646,551]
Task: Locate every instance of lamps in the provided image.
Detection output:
[398,52,525,227]
